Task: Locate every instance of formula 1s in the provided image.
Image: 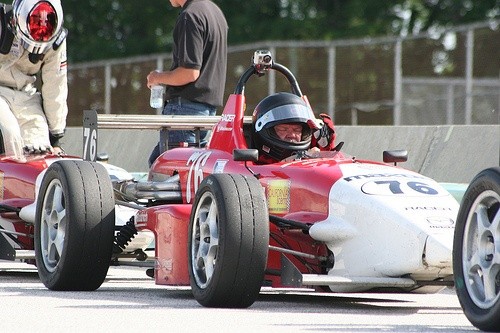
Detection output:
[0,49,461,310]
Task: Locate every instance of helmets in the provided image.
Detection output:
[250,92,318,161]
[9,0,67,63]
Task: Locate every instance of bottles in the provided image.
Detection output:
[149,70,163,108]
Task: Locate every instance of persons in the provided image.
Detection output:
[148,0,228,166]
[251,92,315,167]
[0,1,68,159]
